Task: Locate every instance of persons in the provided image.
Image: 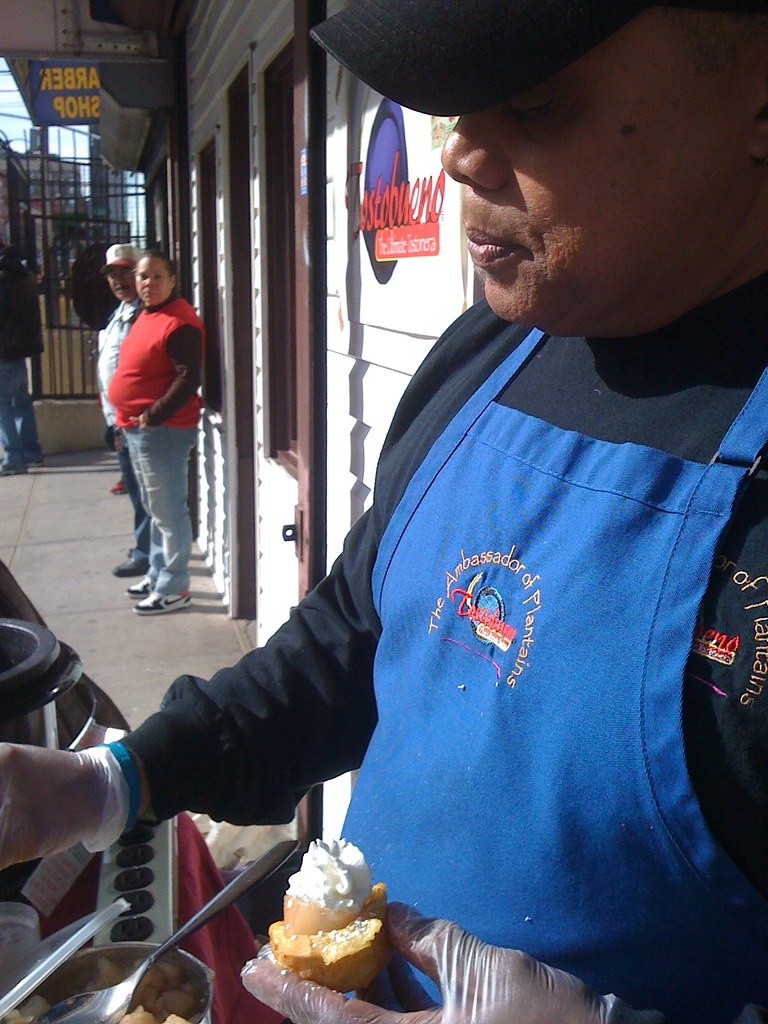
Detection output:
[0,241,44,476]
[0,0,768,1024]
[96,242,204,615]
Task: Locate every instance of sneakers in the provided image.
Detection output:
[124,575,157,599]
[132,590,193,615]
[113,557,150,577]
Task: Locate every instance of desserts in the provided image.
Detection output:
[265,838,392,994]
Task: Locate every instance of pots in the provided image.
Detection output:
[0,615,98,753]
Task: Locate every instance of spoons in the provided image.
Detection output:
[38,840,301,1024]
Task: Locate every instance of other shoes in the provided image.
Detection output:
[0,456,43,467]
[0,466,20,475]
[110,481,127,494]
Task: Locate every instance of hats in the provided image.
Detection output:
[101,244,143,275]
[310,0,648,116]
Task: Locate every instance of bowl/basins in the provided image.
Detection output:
[0,942,214,1024]
